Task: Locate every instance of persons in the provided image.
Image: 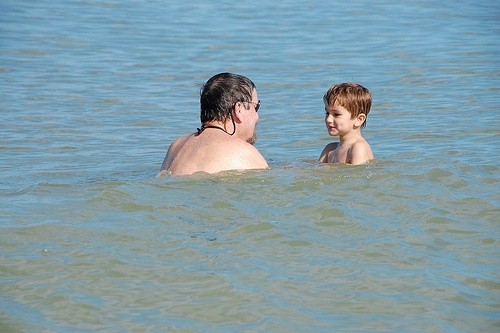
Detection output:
[160,73,271,177]
[319,83,375,165]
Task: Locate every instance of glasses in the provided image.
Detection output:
[234,99,261,112]
